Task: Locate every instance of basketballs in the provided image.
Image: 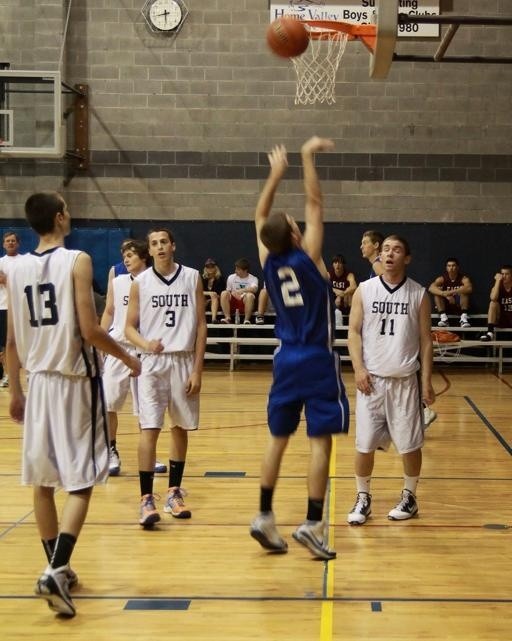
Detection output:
[267,16,311,58]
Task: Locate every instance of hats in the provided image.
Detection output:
[205,258,216,265]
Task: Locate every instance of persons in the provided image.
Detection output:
[124,226,208,526]
[0,231,131,391]
[427,257,475,328]
[479,265,512,341]
[329,254,357,315]
[196,255,277,325]
[346,234,436,526]
[100,239,167,477]
[359,230,384,279]
[5,190,141,619]
[248,134,350,561]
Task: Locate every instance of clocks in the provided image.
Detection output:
[149,0,182,32]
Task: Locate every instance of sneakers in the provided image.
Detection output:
[108,445,121,475]
[480,332,494,340]
[459,317,471,327]
[244,320,251,324]
[139,493,160,526]
[165,486,192,518]
[424,404,437,428]
[438,320,449,326]
[1,375,9,386]
[388,488,418,520]
[293,523,336,559]
[36,562,77,616]
[212,318,231,324]
[251,518,287,551]
[153,461,168,474]
[256,316,264,324]
[347,492,372,525]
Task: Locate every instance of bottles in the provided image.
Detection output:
[235,309,240,324]
